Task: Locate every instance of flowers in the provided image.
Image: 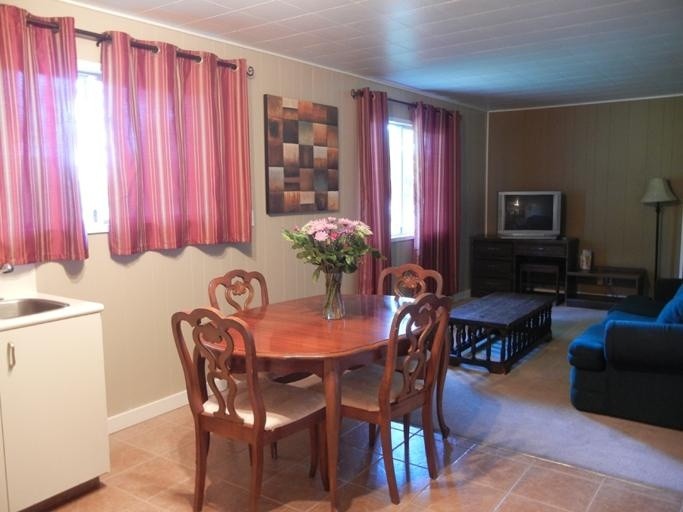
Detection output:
[279,216,388,316]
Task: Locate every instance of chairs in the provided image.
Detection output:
[208,267,313,460]
[348,264,442,448]
[307,293,451,504]
[171,306,329,512]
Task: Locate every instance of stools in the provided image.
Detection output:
[519,262,562,306]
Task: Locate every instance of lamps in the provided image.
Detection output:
[641,177,678,304]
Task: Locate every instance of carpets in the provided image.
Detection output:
[394,297,683,493]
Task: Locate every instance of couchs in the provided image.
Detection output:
[567,282,683,431]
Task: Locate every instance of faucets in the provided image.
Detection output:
[0,260,12,276]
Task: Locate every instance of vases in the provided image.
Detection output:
[323,271,345,320]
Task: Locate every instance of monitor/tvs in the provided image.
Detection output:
[497,191,562,240]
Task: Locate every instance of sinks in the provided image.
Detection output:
[0,298,70,320]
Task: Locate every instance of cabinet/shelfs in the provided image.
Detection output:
[0,313,112,512]
[470,233,645,307]
